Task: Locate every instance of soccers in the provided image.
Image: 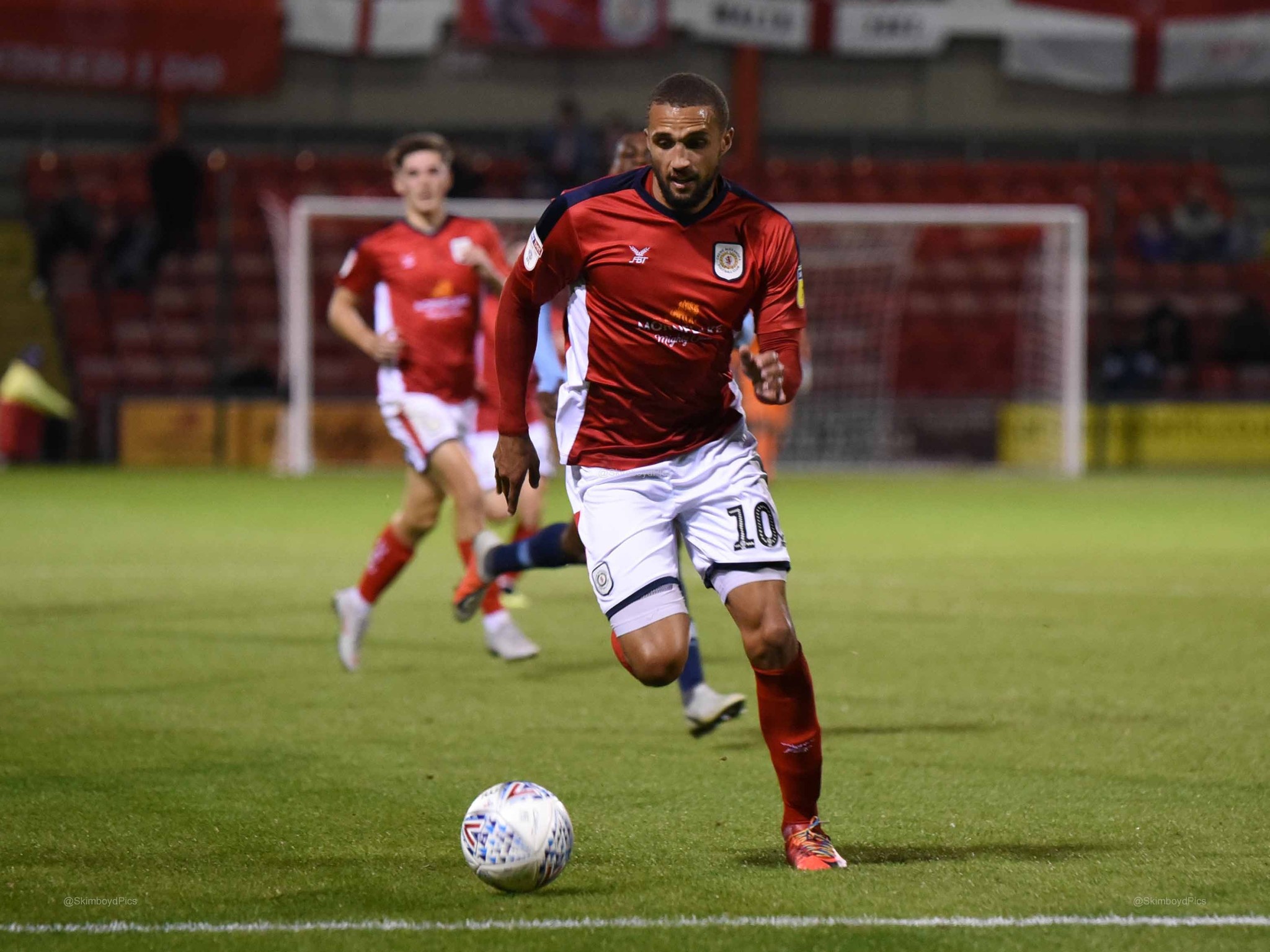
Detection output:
[460,781,575,893]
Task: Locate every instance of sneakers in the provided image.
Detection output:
[331,588,373,669]
[482,609,539,660]
[684,684,746,738]
[453,531,504,623]
[785,816,847,870]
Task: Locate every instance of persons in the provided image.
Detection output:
[466,235,556,610]
[1137,199,1237,266]
[37,199,92,280]
[328,132,542,674]
[107,132,207,285]
[1103,295,1270,403]
[539,97,592,200]
[454,131,748,739]
[493,73,846,872]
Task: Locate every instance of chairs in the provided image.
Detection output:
[26,143,1270,437]
